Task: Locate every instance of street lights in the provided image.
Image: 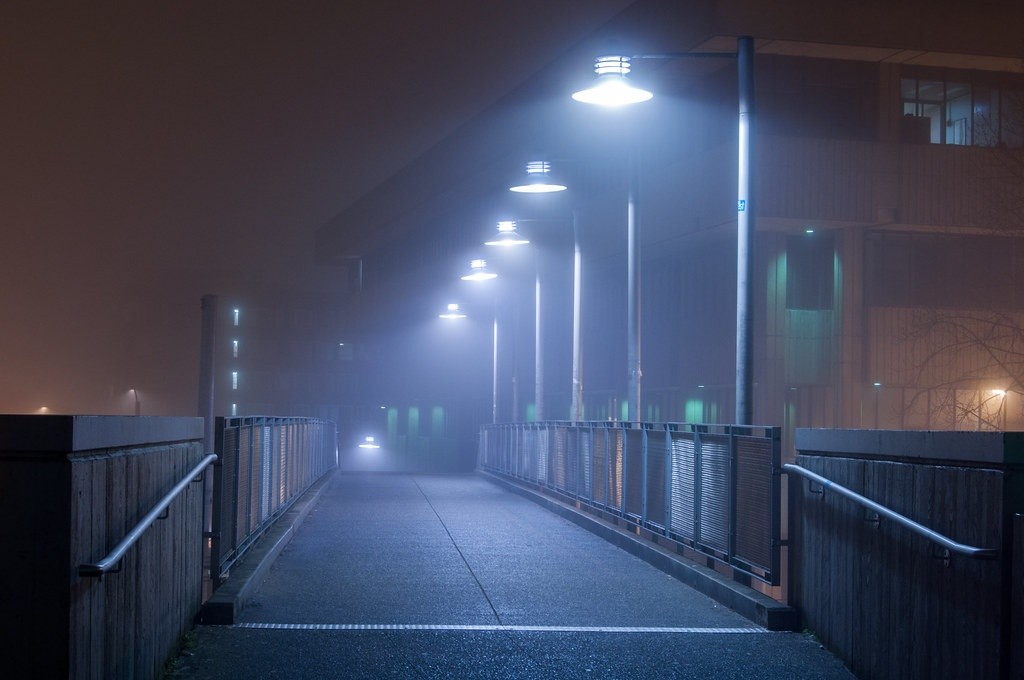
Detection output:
[436,134,644,430]
[572,32,755,432]
